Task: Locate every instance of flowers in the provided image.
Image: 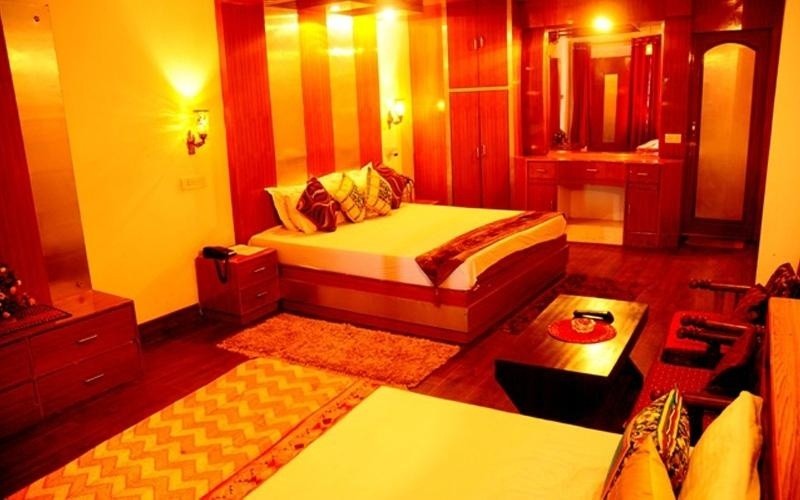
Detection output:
[0,263,35,319]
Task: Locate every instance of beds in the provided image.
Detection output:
[248,162,569,348]
[0,354,800,500]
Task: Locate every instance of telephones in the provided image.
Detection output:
[203,246,236,258]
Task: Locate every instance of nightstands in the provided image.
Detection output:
[195,244,283,329]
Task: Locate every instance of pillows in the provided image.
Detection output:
[263,162,415,234]
[607,436,678,500]
[612,388,692,499]
[677,390,764,500]
[764,262,800,299]
[702,327,758,391]
[730,283,769,325]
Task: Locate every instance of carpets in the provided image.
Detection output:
[217,312,461,389]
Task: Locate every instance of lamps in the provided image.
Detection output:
[387,97,405,129]
[186,109,209,156]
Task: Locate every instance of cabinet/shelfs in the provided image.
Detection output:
[445,87,521,211]
[0,288,144,442]
[443,0,522,90]
[518,160,682,250]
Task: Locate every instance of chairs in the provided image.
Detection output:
[662,262,799,367]
[625,326,770,447]
